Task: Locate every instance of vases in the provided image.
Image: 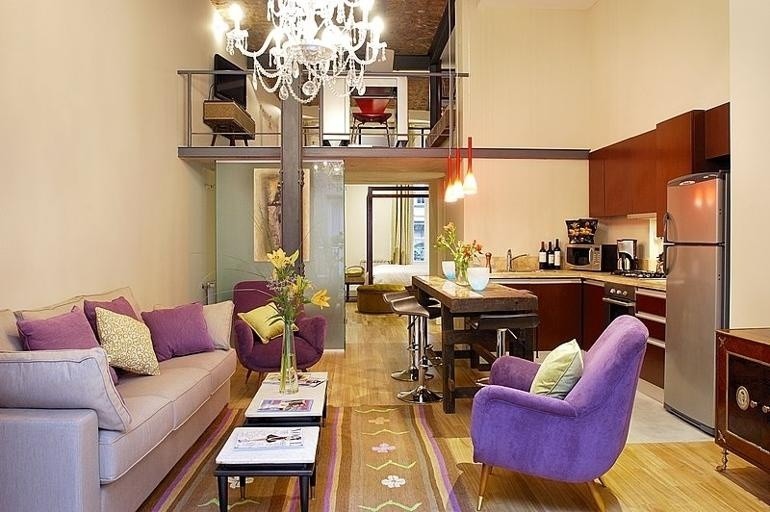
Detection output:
[280,320,300,395]
[452,258,475,286]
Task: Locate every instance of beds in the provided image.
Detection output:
[363,185,429,287]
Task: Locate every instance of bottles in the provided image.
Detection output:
[553,239,561,270]
[546,242,555,269]
[485,252,492,273]
[538,241,549,269]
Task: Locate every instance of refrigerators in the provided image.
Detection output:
[662,169,731,437]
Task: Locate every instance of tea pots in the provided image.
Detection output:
[656,254,665,274]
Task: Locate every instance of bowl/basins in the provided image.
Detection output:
[441,261,456,279]
[467,267,490,290]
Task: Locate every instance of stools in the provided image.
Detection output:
[383,291,433,381]
[464,309,540,391]
[391,297,441,405]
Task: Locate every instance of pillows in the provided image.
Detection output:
[528,336,583,403]
[239,297,301,346]
[16,296,234,386]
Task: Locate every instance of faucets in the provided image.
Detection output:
[506,249,530,269]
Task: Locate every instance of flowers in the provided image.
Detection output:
[434,221,486,278]
[204,200,331,391]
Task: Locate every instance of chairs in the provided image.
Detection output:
[352,95,394,148]
[344,265,366,301]
[469,312,650,512]
[228,276,325,386]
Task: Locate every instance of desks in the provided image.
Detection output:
[411,275,540,415]
[201,99,256,148]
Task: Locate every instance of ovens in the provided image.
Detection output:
[601,296,636,327]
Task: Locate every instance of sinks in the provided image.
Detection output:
[495,269,516,271]
[516,269,533,272]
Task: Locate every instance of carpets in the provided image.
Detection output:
[142,399,475,512]
[625,375,715,444]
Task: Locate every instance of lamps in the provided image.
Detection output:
[444,1,453,205]
[217,0,392,103]
[464,1,478,198]
[452,1,464,200]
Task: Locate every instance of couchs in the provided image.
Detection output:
[0,282,237,512]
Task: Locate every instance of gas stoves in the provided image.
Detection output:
[610,268,666,279]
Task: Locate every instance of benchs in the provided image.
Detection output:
[358,283,406,314]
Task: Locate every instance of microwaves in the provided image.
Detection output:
[563,243,617,272]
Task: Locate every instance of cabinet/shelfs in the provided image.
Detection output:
[588,147,605,218]
[656,109,708,239]
[582,276,611,352]
[603,130,657,215]
[495,279,583,350]
[706,326,769,478]
[633,286,666,390]
[705,103,731,160]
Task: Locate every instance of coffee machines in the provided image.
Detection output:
[616,239,638,270]
